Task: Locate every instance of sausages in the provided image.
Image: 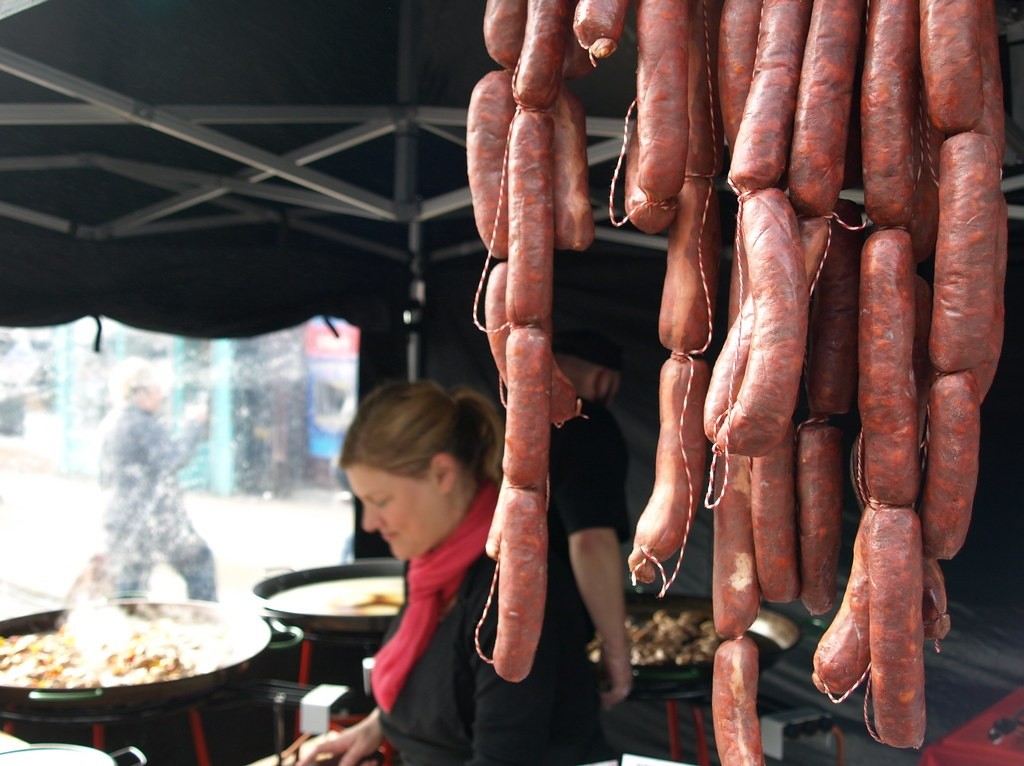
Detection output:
[466,1,1009,766]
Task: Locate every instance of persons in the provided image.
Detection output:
[503,329,633,713]
[97,365,216,602]
[283,380,605,765]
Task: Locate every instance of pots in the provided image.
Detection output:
[622,591,723,684]
[252,559,403,635]
[2,600,272,708]
